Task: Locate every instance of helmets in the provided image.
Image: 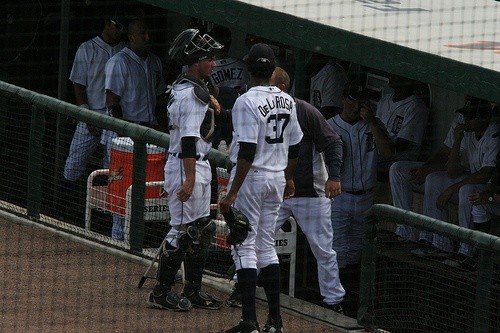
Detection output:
[169,29,216,64]
[247,43,276,71]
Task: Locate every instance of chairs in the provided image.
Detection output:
[85,136,170,248]
[206,167,297,299]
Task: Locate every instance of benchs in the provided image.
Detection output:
[370,179,500,266]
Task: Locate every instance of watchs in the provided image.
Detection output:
[488,193,495,204]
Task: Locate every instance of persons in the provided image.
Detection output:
[63,5,500,333]
[144,29,226,312]
[218,44,304,333]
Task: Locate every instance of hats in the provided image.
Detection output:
[343,80,367,102]
[224,206,250,245]
[455,96,491,120]
[211,26,232,52]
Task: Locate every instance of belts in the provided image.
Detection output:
[346,190,367,195]
[170,152,210,162]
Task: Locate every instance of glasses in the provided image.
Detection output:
[110,19,128,33]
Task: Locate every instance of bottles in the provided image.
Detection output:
[216,167,230,186]
[218,186,227,219]
[218,140,228,153]
[233,274,238,289]
[215,215,230,247]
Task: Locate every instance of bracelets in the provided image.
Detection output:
[107,104,123,119]
[456,181,464,188]
[79,104,90,109]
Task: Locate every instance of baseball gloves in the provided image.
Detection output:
[223,206,249,245]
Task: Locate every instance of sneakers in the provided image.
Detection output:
[411,239,438,255]
[149,291,191,311]
[225,319,260,333]
[226,295,242,308]
[260,314,286,333]
[442,253,476,270]
[378,233,416,250]
[181,290,224,309]
